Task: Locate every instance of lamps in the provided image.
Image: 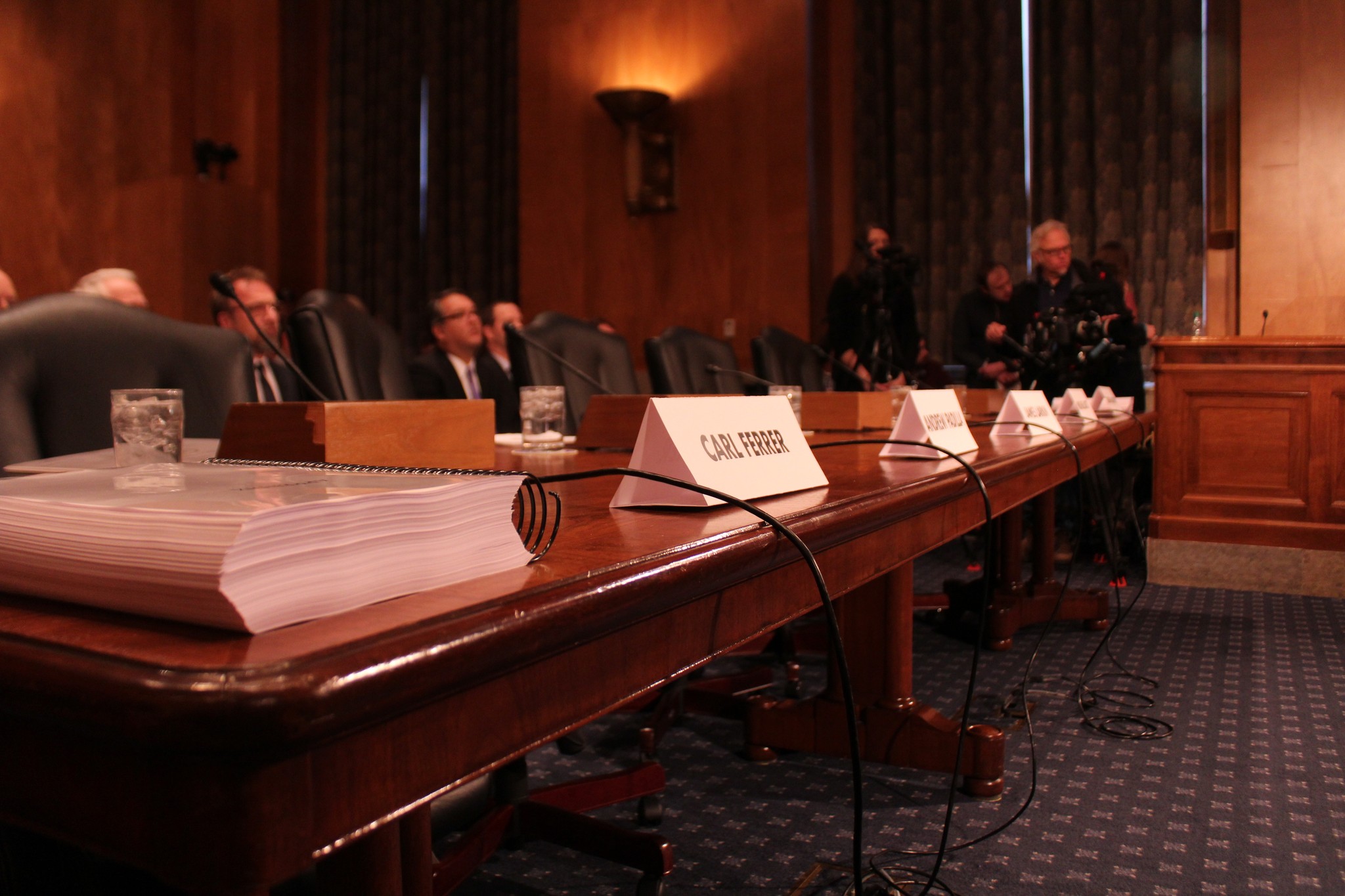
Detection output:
[593,86,676,214]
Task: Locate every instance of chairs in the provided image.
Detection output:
[645,328,743,394]
[0,293,250,476]
[286,291,413,402]
[752,326,827,394]
[525,313,638,435]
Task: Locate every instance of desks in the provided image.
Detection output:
[0,415,1151,896]
[1150,334,1345,550]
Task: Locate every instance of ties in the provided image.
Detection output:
[467,370,481,400]
[253,360,276,402]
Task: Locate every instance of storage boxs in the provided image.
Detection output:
[802,391,906,429]
[575,394,744,449]
[216,397,495,469]
[956,389,1009,422]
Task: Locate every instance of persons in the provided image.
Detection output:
[827,218,1155,561]
[0,262,305,403]
[415,288,525,435]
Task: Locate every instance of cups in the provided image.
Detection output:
[768,386,801,429]
[945,384,967,419]
[110,388,185,466]
[890,385,918,429]
[519,385,567,450]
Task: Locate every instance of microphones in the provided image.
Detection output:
[705,363,774,387]
[211,272,328,401]
[863,352,937,391]
[503,323,613,393]
[810,346,880,393]
[1261,310,1269,336]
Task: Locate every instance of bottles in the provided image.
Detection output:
[1192,312,1201,336]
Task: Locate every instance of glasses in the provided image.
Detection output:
[1036,243,1072,258]
[230,300,279,316]
[438,308,477,324]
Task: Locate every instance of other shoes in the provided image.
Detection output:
[1020,536,1032,558]
[1055,543,1073,563]
